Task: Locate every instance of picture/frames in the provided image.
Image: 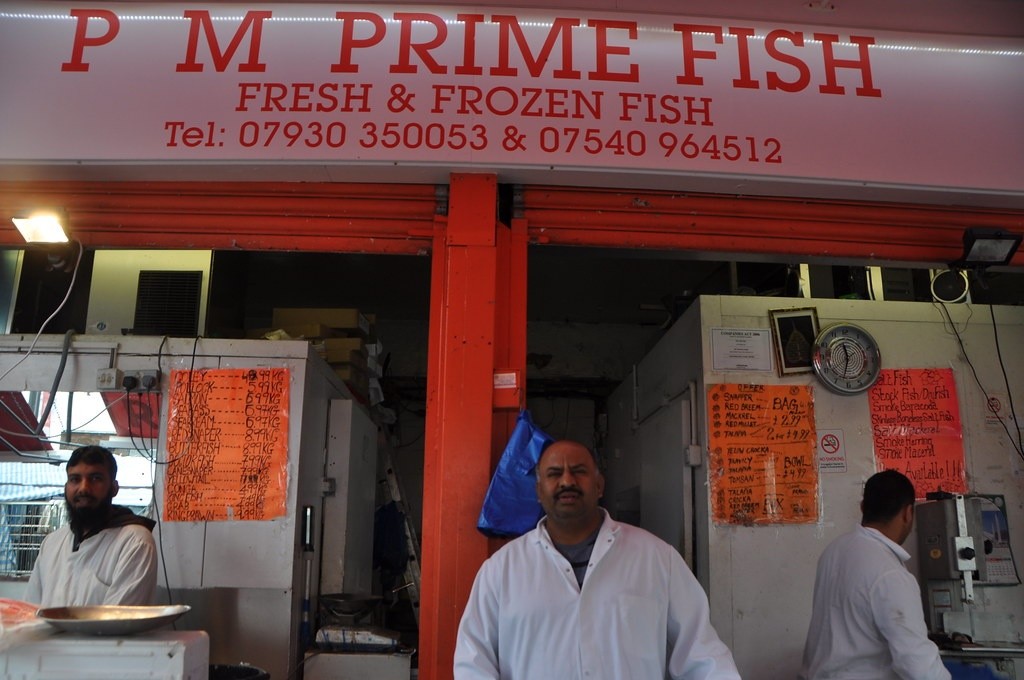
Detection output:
[768,306,821,379]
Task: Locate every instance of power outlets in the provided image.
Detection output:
[122,370,158,390]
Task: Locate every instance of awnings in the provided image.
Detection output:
[795,469,952,680]
[0,391,58,465]
[98,393,161,452]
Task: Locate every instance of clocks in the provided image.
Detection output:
[812,321,882,396]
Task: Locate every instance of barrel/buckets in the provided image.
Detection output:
[319,594,359,625]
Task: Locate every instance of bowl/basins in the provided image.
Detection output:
[36,605,192,638]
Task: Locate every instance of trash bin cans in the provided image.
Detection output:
[209,664,270,680]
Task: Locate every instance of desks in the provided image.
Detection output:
[302,648,415,680]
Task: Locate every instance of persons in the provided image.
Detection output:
[452,441,743,680]
[21,446,159,608]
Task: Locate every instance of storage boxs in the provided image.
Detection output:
[246,307,384,406]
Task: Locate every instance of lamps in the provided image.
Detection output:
[948,227,1024,270]
[12,205,70,243]
